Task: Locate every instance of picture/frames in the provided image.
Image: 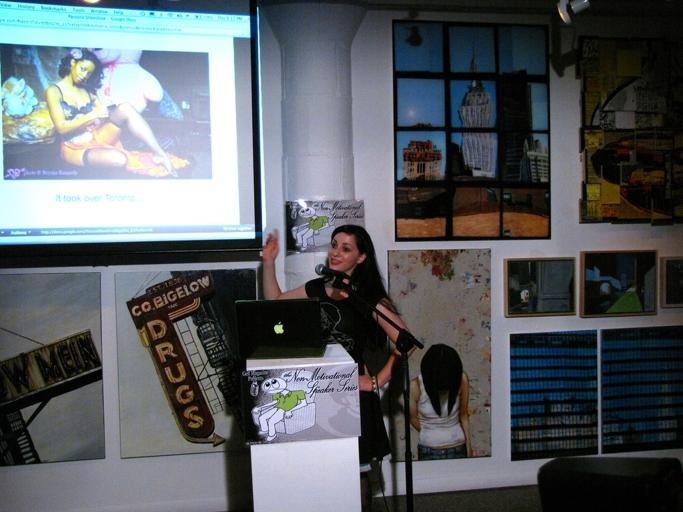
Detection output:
[503,250,682,323]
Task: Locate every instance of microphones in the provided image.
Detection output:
[314,264,352,280]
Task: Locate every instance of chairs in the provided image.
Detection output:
[537,457,682,512]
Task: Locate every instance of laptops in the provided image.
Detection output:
[234,297,330,359]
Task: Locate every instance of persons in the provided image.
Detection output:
[409,344,472,460]
[46,48,179,177]
[263,225,416,511]
[519,277,537,312]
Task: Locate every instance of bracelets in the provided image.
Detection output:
[372,376,377,393]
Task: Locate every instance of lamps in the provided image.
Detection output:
[557,0,594,27]
